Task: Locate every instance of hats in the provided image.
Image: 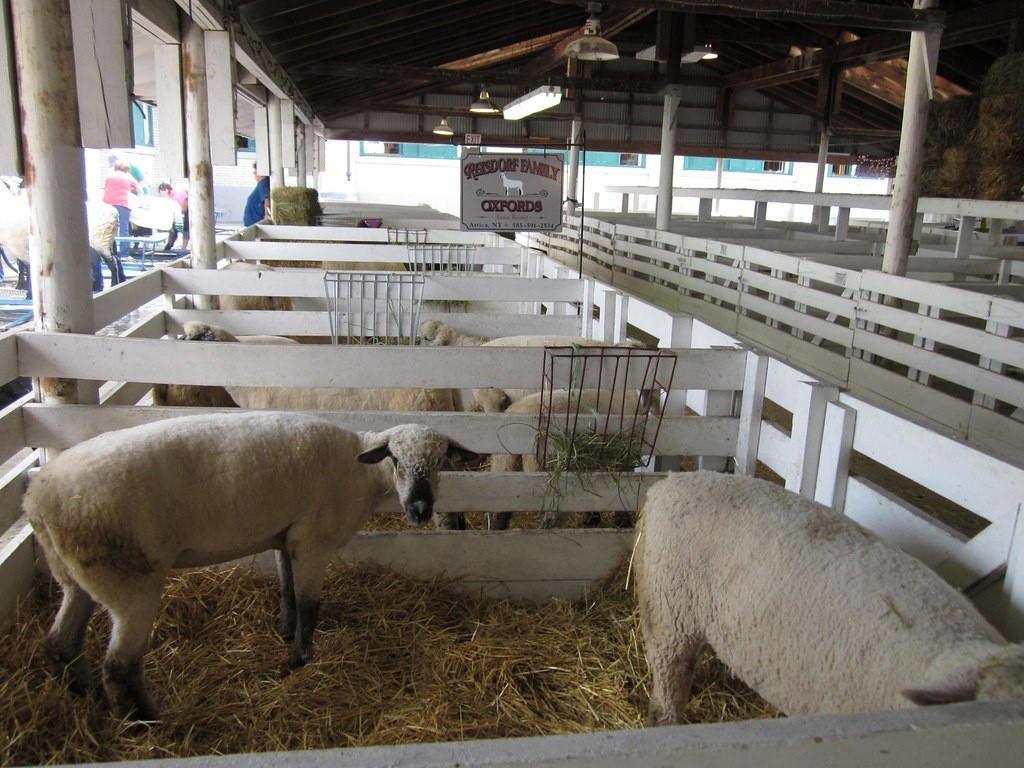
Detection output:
[108,154,118,169]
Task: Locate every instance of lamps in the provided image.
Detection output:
[469,82,500,114]
[500,82,563,119]
[563,1,623,61]
[432,114,454,135]
[702,42,718,60]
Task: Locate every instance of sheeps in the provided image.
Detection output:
[17,409,478,739]
[636,467,1024,727]
[151,261,662,531]
[0,175,126,298]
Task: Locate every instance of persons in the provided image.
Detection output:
[243,161,271,227]
[102,160,144,263]
[106,154,152,250]
[157,181,190,254]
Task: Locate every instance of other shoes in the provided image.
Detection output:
[121,255,134,262]
[132,248,138,255]
